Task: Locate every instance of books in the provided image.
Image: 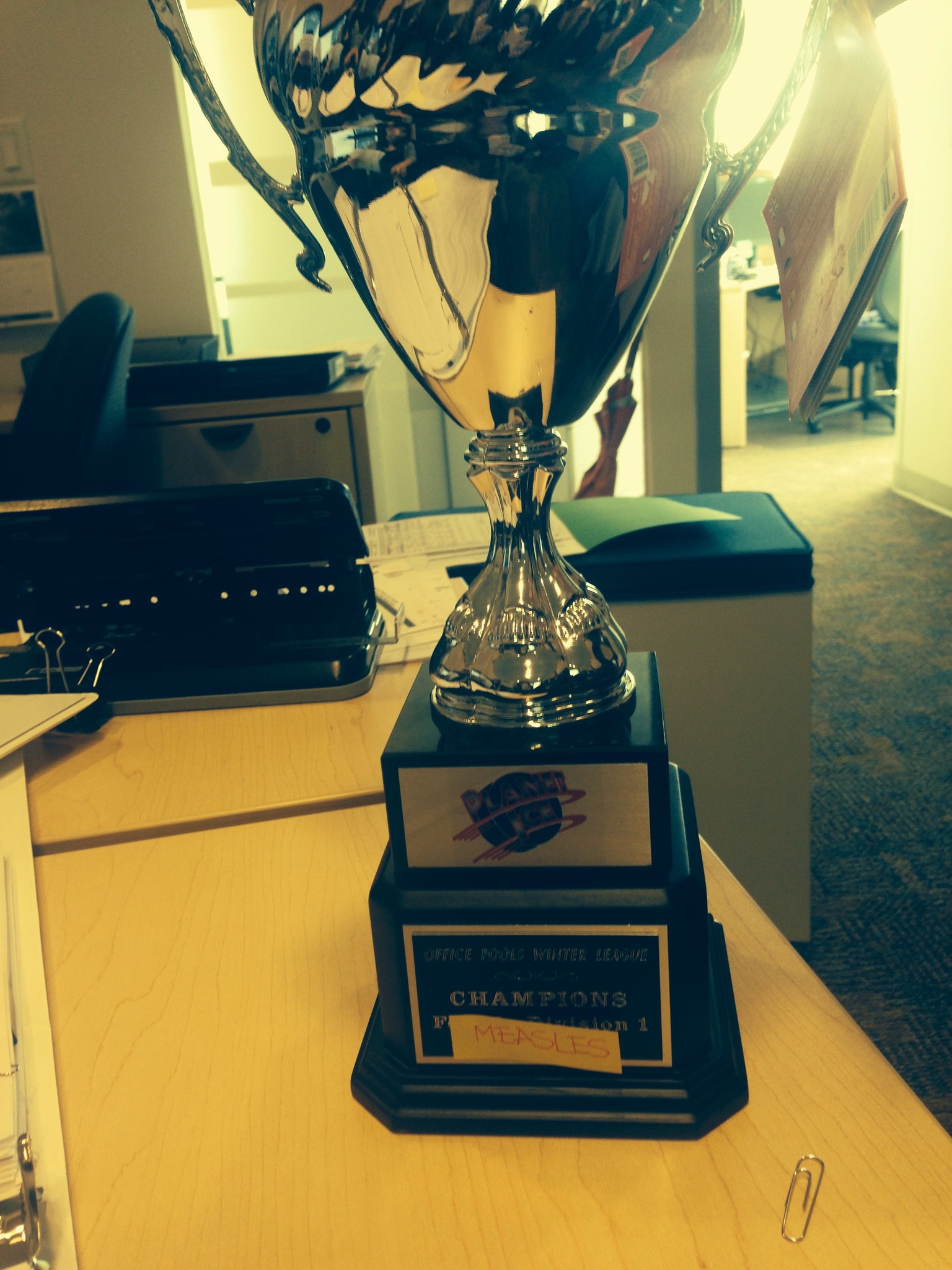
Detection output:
[761,1,909,423]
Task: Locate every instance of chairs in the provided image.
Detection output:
[1,291,135,501]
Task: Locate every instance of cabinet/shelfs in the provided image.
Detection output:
[130,341,420,525]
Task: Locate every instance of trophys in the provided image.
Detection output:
[147,1,827,1142]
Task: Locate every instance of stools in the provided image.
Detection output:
[807,327,898,433]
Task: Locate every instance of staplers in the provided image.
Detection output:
[0,475,388,717]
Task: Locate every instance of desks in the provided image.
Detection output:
[22,657,952,1270]
[719,266,787,449]
